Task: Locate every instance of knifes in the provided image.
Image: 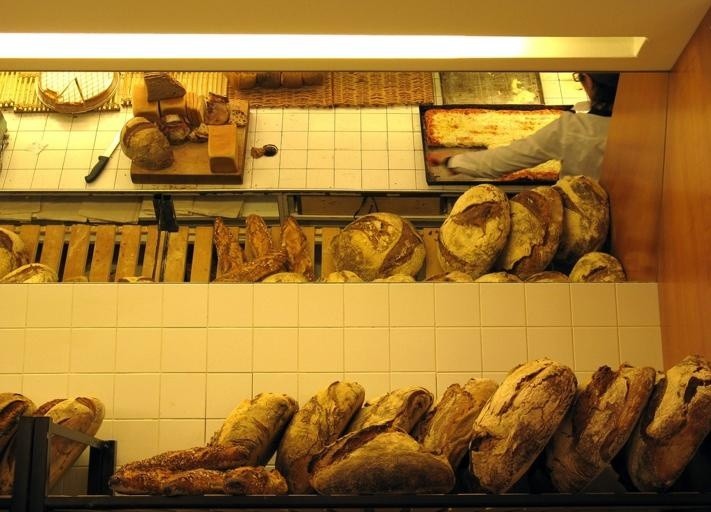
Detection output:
[84,130,122,184]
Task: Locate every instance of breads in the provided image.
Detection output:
[120,71,325,173]
[1,226,155,283]
[107,353,710,494]
[0,392,105,496]
[210,175,627,284]
[423,108,564,181]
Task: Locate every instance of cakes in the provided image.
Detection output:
[37,72,118,114]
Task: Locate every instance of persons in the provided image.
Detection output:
[427,71,619,183]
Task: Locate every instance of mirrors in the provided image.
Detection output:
[0,70,670,283]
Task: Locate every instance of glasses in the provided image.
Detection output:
[573,72,583,82]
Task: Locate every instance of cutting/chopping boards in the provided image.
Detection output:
[129,99,250,186]
